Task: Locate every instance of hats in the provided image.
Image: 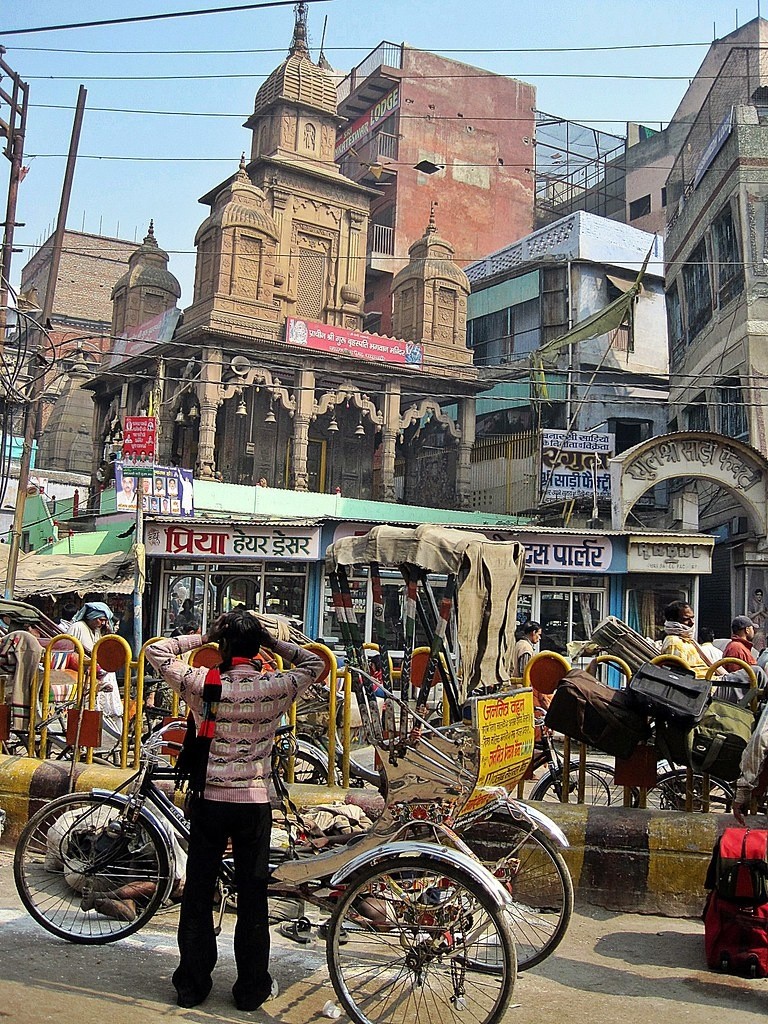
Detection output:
[731,614,759,632]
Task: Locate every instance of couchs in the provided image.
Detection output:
[20,637,108,703]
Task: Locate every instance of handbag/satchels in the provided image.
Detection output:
[626,660,712,723]
[663,686,757,782]
[544,667,652,760]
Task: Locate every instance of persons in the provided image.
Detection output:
[140,478,151,494]
[143,497,148,511]
[662,600,768,826]
[146,435,153,444]
[749,588,768,628]
[147,609,325,1012]
[167,479,177,495]
[178,467,193,516]
[101,452,117,492]
[179,599,196,622]
[118,477,138,504]
[66,601,124,716]
[155,478,165,495]
[125,434,132,443]
[150,498,159,512]
[94,461,108,513]
[509,621,554,780]
[123,450,153,466]
[172,592,181,614]
[162,499,170,512]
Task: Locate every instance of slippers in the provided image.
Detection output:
[280,914,319,943]
[318,917,350,945]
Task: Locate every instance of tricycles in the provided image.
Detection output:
[0,523,768,1022]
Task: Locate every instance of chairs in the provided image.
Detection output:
[312,654,384,728]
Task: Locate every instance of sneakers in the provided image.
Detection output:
[252,977,279,1010]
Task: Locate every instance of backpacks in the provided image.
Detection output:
[700,826,768,977]
